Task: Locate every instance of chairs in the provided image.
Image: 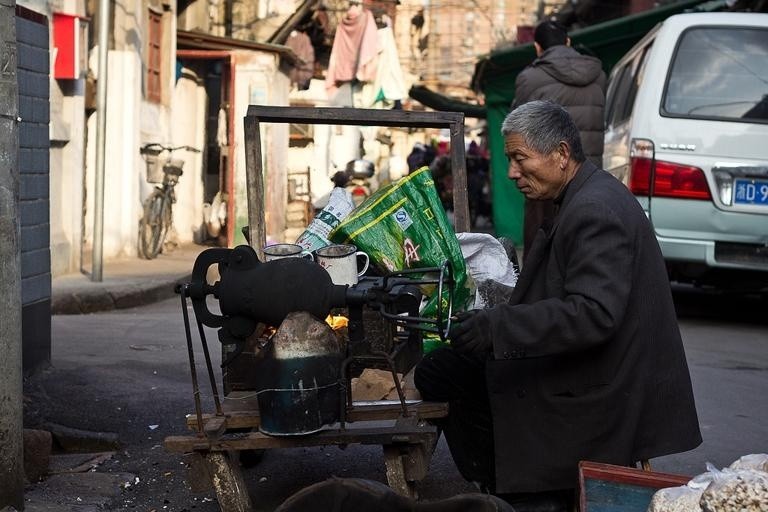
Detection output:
[285,179,312,227]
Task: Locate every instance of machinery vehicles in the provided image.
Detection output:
[165,103,474,512]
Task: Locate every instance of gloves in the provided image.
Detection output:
[449,309,491,353]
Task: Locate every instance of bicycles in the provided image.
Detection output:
[141,143,202,259]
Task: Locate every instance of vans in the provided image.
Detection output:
[600,11,767,295]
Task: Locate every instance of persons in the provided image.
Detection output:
[412,94,705,512]
[404,139,493,231]
[508,17,608,268]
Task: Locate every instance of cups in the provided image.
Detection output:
[315,244,370,289]
[262,243,314,262]
